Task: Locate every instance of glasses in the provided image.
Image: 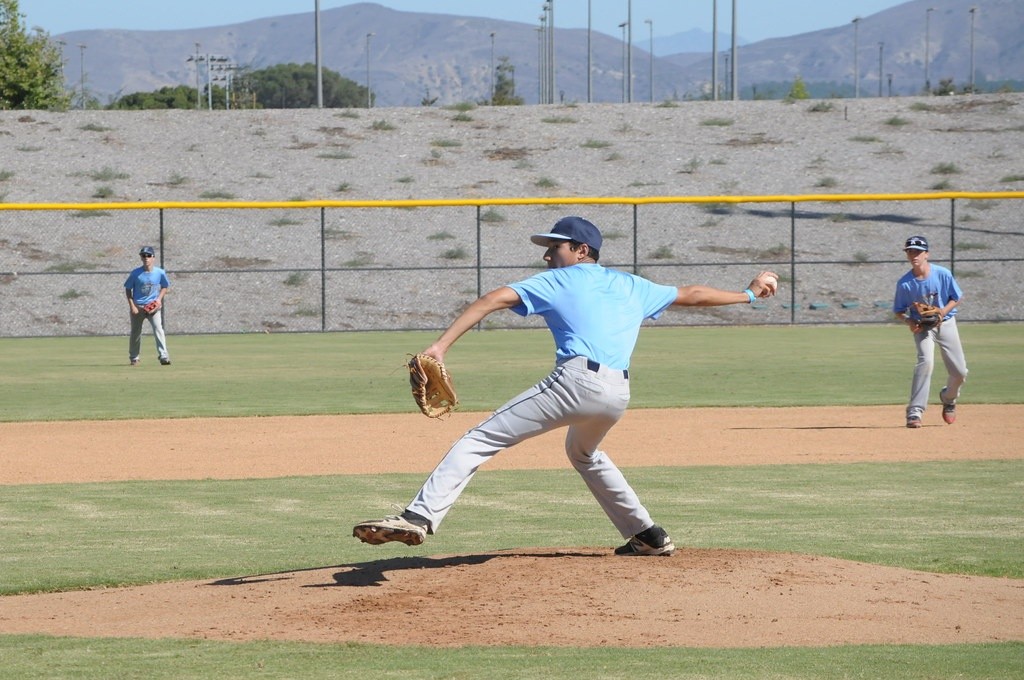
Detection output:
[140,254,152,258]
[906,241,927,247]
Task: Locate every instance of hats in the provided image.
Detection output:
[530,217,602,253]
[903,236,927,252]
[139,247,154,257]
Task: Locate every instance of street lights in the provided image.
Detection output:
[723,52,730,100]
[643,19,656,103]
[365,32,376,109]
[618,22,627,103]
[76,43,86,110]
[968,6,976,95]
[924,8,938,94]
[876,41,885,97]
[534,1,558,106]
[851,15,860,99]
[195,40,204,110]
[488,32,496,103]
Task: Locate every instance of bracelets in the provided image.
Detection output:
[902,315,910,323]
[742,289,756,304]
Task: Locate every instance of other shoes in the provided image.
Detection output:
[161,358,170,365]
[131,360,137,365]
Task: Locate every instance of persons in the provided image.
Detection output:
[123,246,172,365]
[893,236,968,428]
[352,216,779,557]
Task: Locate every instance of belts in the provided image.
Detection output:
[588,360,629,379]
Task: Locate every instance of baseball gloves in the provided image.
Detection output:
[910,300,943,334]
[142,300,160,317]
[408,352,458,420]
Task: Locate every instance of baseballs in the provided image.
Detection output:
[767,277,777,289]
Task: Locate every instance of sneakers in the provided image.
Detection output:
[352,514,429,545]
[616,527,675,556]
[942,386,956,424]
[907,416,921,428]
